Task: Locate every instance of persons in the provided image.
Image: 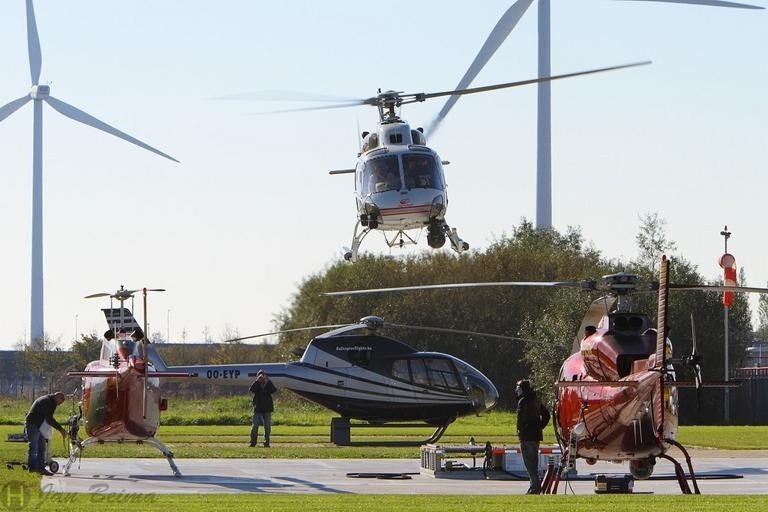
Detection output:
[248,370,277,448]
[25,392,68,476]
[366,159,434,191]
[515,380,550,494]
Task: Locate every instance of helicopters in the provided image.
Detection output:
[324,272,766,500]
[96,305,498,445]
[260,61,652,263]
[62,290,186,481]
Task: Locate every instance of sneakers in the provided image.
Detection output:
[29,467,53,476]
